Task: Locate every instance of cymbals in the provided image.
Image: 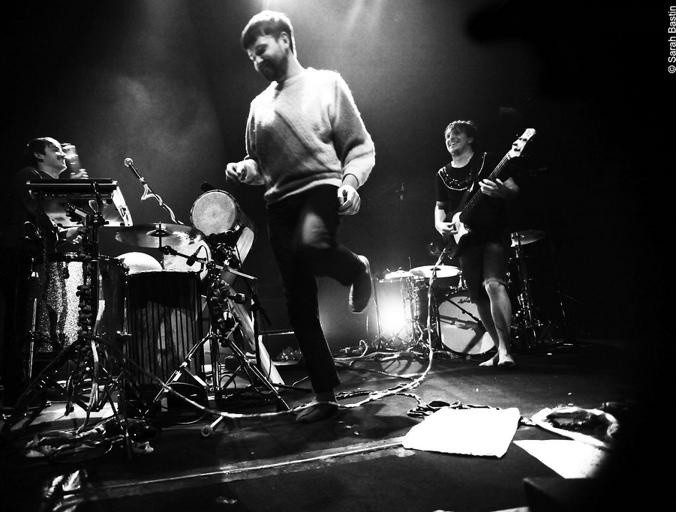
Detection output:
[511,229,546,247]
[410,266,462,280]
[115,222,189,249]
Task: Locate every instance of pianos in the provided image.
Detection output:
[25,178,131,228]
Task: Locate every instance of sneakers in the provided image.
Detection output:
[296,400,338,420]
[350,255,370,312]
[479,353,516,366]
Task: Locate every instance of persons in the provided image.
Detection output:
[222,8,378,426]
[11,135,89,410]
[432,119,522,369]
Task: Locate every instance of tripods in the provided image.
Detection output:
[0,196,145,428]
[140,246,291,421]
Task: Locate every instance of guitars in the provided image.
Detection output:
[445,127,535,258]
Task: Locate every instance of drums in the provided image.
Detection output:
[189,189,255,242]
[115,253,162,274]
[433,288,498,356]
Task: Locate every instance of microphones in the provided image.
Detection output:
[124,158,145,184]
